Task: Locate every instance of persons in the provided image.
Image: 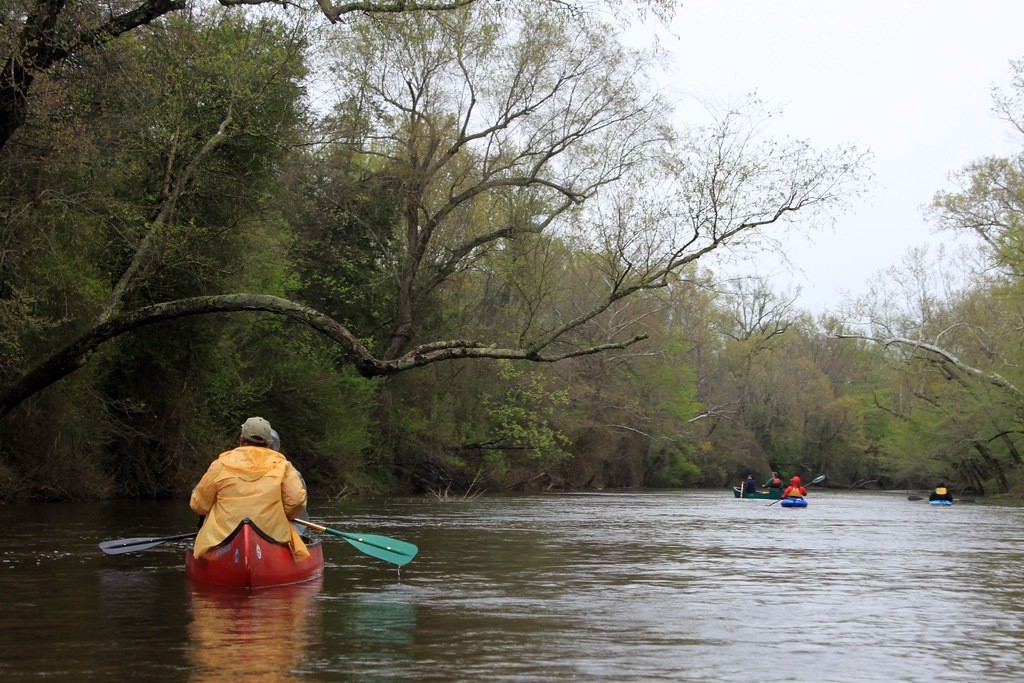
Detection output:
[762,472,783,493]
[190,417,310,562]
[929,483,953,502]
[781,476,808,499]
[743,475,756,494]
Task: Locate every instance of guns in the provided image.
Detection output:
[291,518,418,567]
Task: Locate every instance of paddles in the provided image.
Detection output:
[98,531,198,556]
[908,495,976,503]
[765,474,826,506]
[740,481,744,498]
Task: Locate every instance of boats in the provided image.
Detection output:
[929,500,951,506]
[734,486,783,499]
[185,518,325,591]
[781,497,808,508]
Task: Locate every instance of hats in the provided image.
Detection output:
[792,476,800,488]
[937,483,945,487]
[747,475,752,479]
[774,472,778,475]
[241,417,280,451]
